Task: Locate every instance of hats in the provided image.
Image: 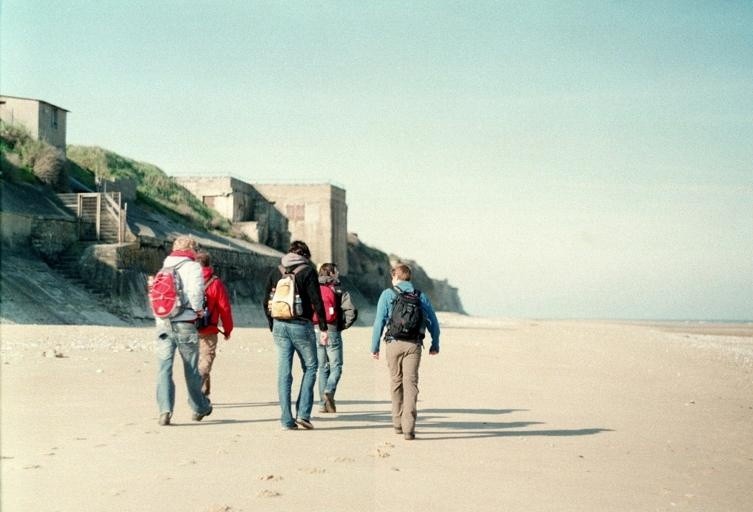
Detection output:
[173,238,194,251]
[288,241,310,259]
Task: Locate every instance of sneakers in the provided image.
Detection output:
[281,424,297,430]
[192,405,212,421]
[325,394,336,413]
[296,416,313,429]
[159,413,168,425]
[319,408,327,413]
[394,427,402,433]
[405,433,414,440]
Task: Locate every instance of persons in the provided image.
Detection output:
[195,252,234,405]
[370,263,440,440]
[148,236,213,426]
[312,263,358,413]
[263,240,329,430]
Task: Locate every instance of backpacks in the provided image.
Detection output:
[312,283,338,324]
[151,259,193,317]
[268,264,308,320]
[386,286,422,340]
[194,274,218,329]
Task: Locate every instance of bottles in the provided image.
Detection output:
[335,287,343,295]
[176,289,184,309]
[146,274,153,297]
[202,306,209,326]
[267,288,275,313]
[293,294,302,315]
